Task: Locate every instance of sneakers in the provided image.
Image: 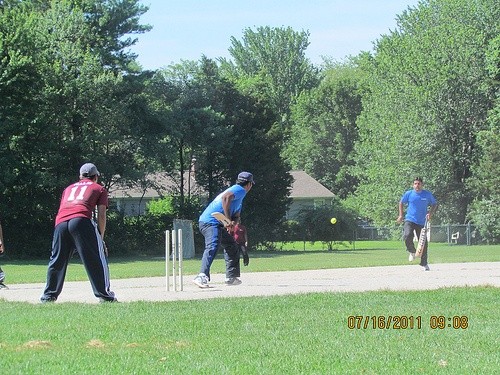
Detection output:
[192,275,208,287]
[225,277,241,285]
[0,284,9,289]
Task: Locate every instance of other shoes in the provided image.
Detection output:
[408,253,416,262]
[421,265,429,271]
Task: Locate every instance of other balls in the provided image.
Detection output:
[331,218,336,224]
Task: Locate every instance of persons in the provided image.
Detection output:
[396,177,436,271]
[233,217,249,266]
[0,222,9,290]
[39,163,119,303]
[192,171,255,290]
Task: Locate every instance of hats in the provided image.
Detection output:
[80,163,100,177]
[238,172,255,184]
